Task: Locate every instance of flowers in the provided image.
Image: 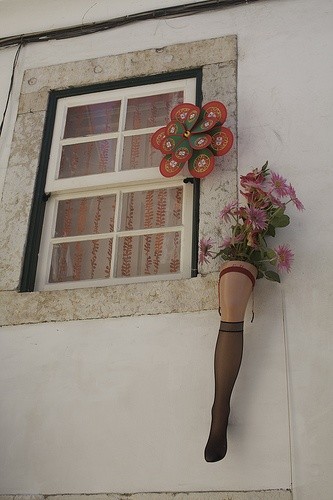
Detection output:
[198,160,304,283]
